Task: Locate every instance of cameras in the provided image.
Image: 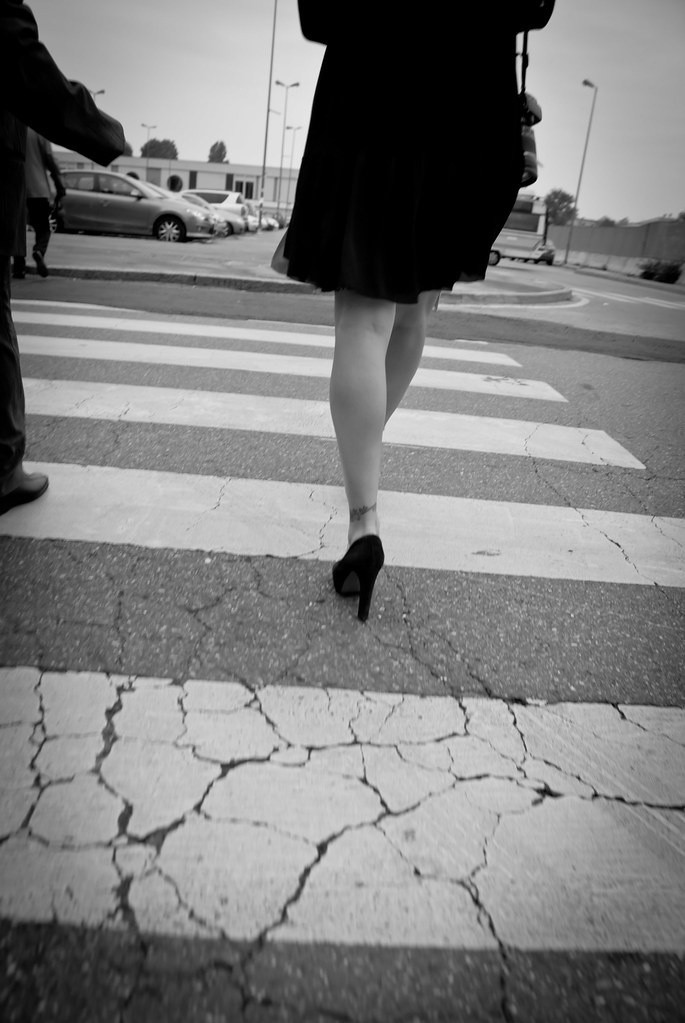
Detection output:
[519,92,542,188]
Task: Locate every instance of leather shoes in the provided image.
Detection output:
[0,473,48,516]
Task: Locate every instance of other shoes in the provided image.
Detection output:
[13,269,26,279]
[32,250,49,278]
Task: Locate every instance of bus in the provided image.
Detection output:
[486,192,548,267]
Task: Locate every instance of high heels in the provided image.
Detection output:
[332,535,384,620]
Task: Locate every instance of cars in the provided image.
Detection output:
[145,189,288,239]
[531,239,555,265]
[50,168,219,244]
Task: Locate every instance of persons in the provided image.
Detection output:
[270,0,556,622]
[11,127,67,280]
[0,0,126,511]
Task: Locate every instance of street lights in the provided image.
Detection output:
[274,80,300,219]
[88,90,107,169]
[285,124,302,221]
[141,123,157,182]
[562,78,600,264]
